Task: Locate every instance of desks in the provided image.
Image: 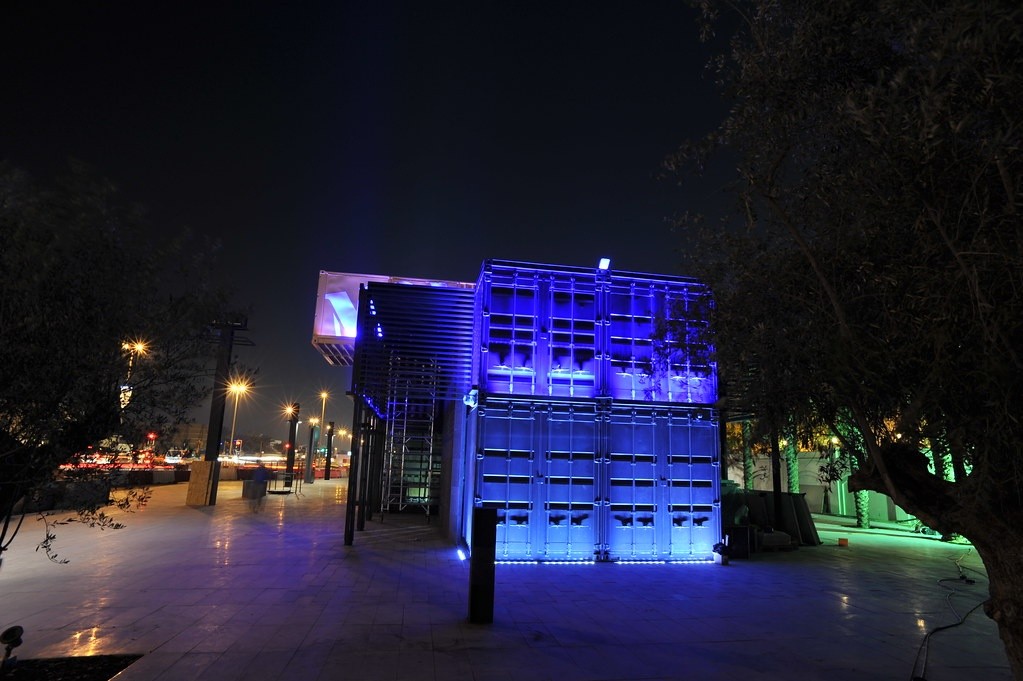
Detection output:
[721,520,751,560]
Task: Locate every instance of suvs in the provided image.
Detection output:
[164,450,182,466]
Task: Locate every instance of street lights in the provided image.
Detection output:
[348,434,351,451]
[122,342,142,379]
[316,392,327,467]
[296,421,302,445]
[230,384,247,455]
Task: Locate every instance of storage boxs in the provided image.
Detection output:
[713,552,729,566]
